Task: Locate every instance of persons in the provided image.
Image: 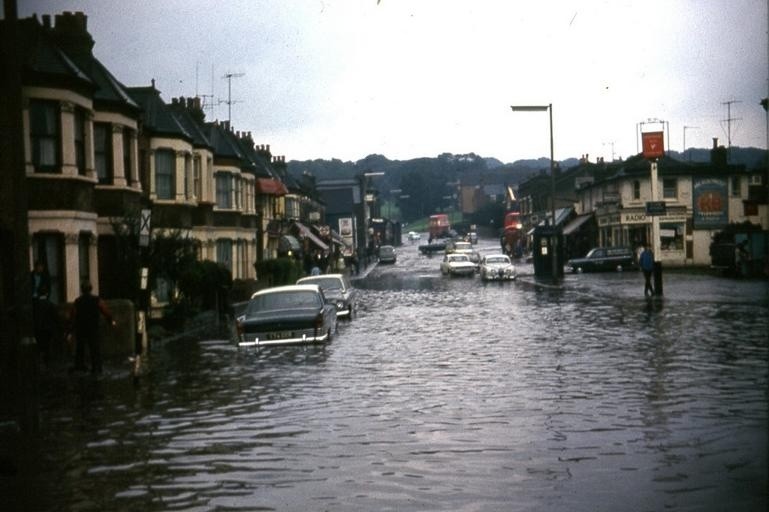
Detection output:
[66,281,116,376]
[195,273,217,312]
[639,243,654,298]
[31,258,51,341]
[219,261,235,321]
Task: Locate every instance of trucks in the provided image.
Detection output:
[428,214,480,244]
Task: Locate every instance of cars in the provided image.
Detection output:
[378,245,397,263]
[467,233,477,244]
[441,238,516,283]
[237,284,339,348]
[296,272,358,323]
[418,240,446,254]
[406,231,423,242]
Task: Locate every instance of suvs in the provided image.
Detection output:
[565,246,635,275]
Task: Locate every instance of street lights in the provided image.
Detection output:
[508,100,559,283]
